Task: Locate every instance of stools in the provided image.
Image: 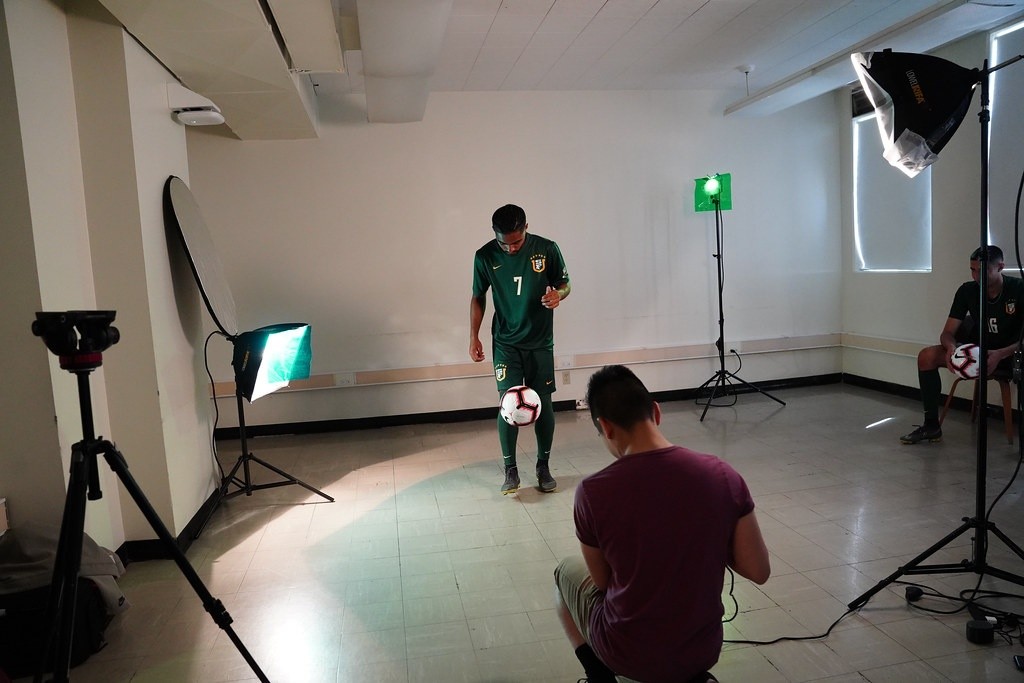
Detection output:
[936,375,1015,446]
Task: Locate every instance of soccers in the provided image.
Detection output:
[498,385,541,428]
[950,344,979,380]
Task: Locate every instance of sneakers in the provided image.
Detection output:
[900,424,943,444]
[536,459,557,492]
[500,463,520,494]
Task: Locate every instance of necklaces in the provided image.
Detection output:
[988,289,1001,304]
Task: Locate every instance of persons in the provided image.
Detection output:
[468,205,571,495]
[898,245,1023,446]
[553,366,774,683]
